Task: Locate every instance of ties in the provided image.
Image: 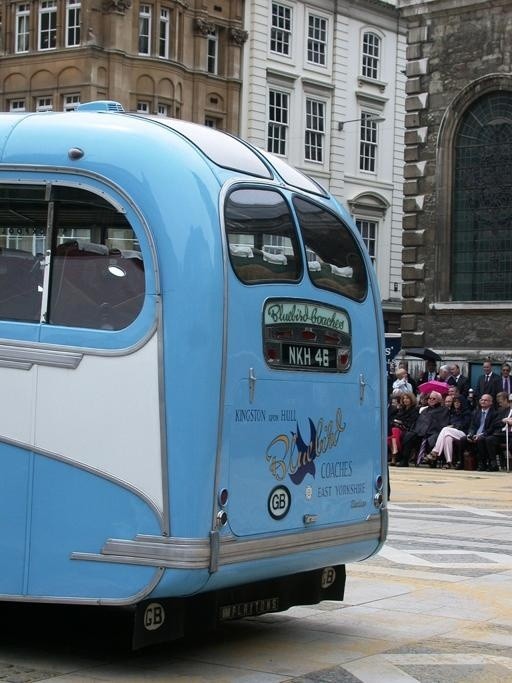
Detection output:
[484,376,489,389]
[476,412,486,434]
[505,379,508,393]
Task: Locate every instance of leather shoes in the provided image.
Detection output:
[473,463,498,471]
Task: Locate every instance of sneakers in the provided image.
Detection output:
[390,450,409,467]
[422,451,465,470]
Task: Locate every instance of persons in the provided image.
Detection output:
[389,362,512,472]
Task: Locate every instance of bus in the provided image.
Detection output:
[0,101,391,651]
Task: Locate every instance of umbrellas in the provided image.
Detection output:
[402,347,441,361]
